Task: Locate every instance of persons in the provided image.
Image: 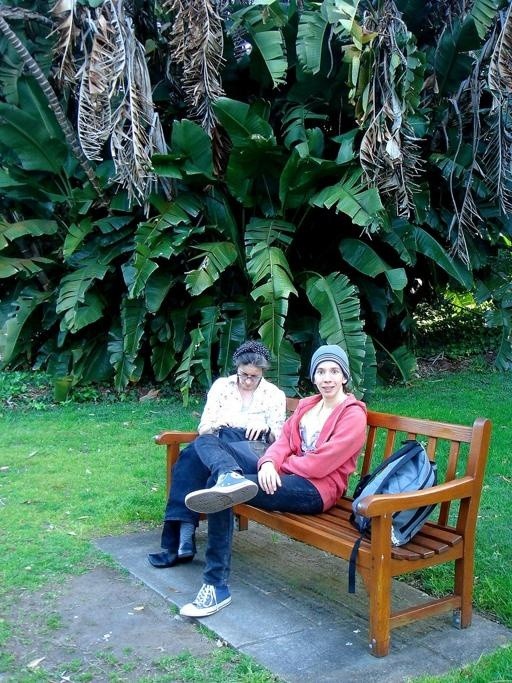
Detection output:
[179,345,366,616]
[148,342,286,568]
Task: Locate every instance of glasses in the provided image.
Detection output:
[237,369,261,380]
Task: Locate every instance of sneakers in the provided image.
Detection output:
[178,540,197,557]
[184,471,259,515]
[146,544,197,568]
[179,583,232,617]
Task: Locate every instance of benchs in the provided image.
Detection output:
[158,398,494,668]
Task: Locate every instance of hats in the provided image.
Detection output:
[309,344,350,383]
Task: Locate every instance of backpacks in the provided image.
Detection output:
[349,439,438,548]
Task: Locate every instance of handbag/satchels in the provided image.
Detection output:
[219,426,268,462]
